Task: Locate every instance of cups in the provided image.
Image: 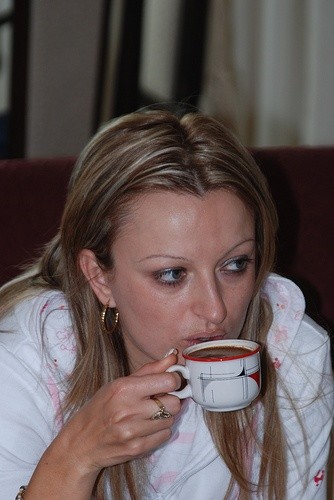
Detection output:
[165,339,262,412]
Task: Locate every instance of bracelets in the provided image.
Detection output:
[15,484,26,500]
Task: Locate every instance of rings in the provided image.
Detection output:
[151,397,171,420]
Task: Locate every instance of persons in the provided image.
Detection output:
[1,107,334,500]
